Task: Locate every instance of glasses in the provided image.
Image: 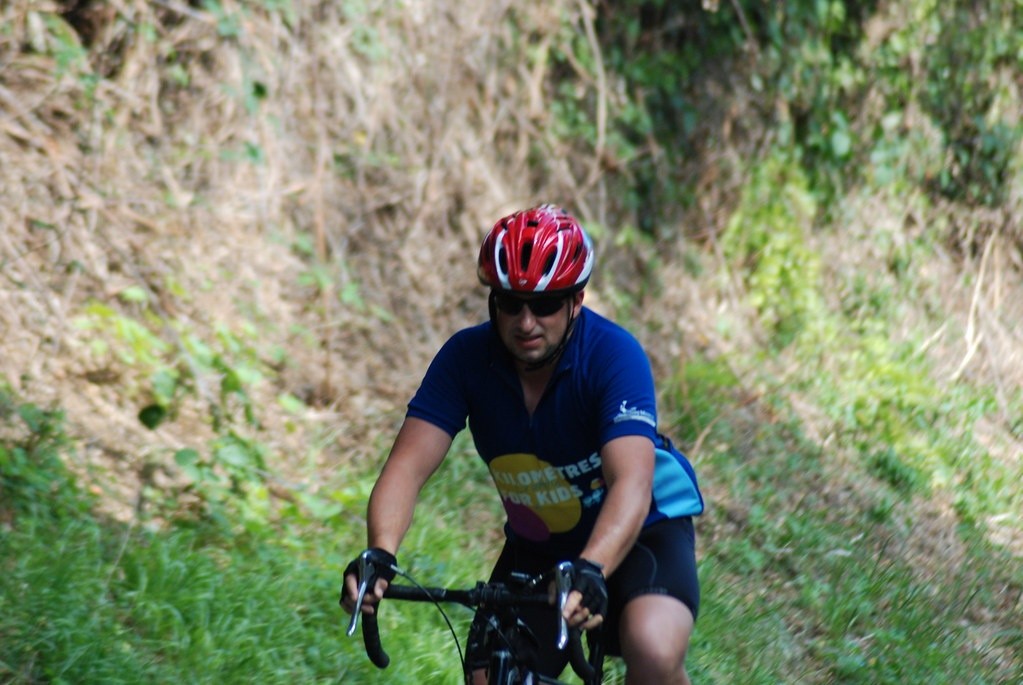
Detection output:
[487,291,576,317]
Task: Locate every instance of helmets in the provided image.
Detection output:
[475,202,595,296]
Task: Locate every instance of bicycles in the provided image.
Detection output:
[344,546,610,685]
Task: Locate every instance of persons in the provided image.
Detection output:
[339,204,705,685]
